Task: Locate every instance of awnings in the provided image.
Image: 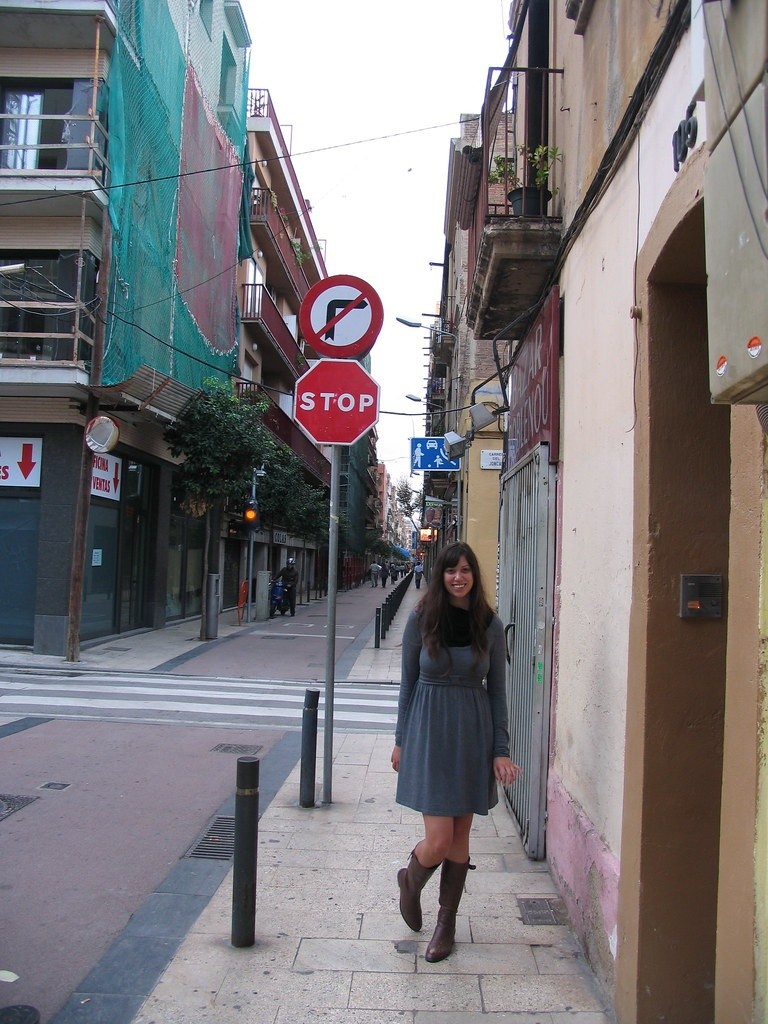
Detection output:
[77,366,205,430]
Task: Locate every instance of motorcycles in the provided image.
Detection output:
[270,577,295,616]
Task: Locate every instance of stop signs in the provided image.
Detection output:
[292,358,381,446]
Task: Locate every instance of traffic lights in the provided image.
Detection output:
[243,501,258,524]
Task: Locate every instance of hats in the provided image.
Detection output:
[417,561,420,565]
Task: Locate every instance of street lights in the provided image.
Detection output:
[246,468,267,623]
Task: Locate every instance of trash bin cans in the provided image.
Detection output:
[206,573,222,640]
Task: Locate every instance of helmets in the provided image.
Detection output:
[286,558,295,564]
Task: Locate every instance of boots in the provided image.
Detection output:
[397,839,443,932]
[425,856,476,963]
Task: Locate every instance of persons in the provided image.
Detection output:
[368,561,408,588]
[415,562,424,589]
[391,541,523,963]
[271,558,298,616]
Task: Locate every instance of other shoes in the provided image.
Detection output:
[291,614,294,617]
